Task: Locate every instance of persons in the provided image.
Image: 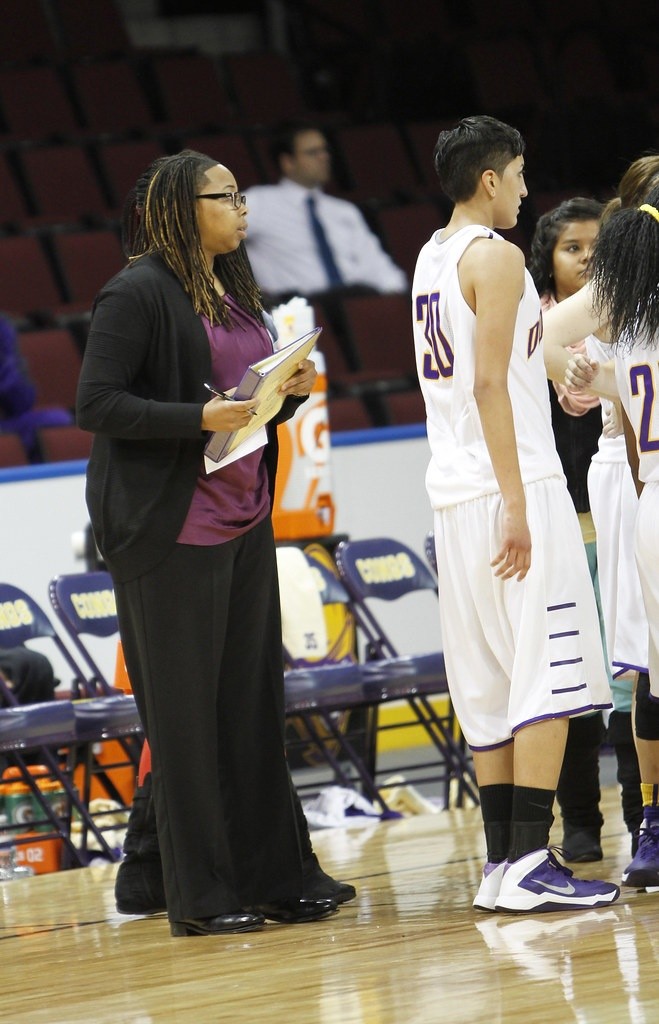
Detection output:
[241,128,405,394]
[0,317,71,448]
[528,154,659,891]
[78,151,356,938]
[414,116,619,912]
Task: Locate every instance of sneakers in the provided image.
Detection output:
[474,915,513,954]
[497,912,620,983]
[305,785,403,827]
[621,817,659,886]
[473,858,509,911]
[495,846,620,912]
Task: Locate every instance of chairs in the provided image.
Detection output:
[0,0,658,466]
[0,532,481,870]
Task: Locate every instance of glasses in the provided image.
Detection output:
[195,192,246,208]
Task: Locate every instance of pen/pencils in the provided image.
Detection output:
[204,383,258,416]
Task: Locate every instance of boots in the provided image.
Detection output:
[608,710,643,858]
[556,711,604,863]
[302,854,355,903]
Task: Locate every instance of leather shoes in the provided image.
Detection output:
[240,899,335,923]
[171,909,266,936]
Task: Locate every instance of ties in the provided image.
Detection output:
[307,196,342,288]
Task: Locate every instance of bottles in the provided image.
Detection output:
[0,814,16,882]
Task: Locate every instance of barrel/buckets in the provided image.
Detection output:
[271,352,334,540]
